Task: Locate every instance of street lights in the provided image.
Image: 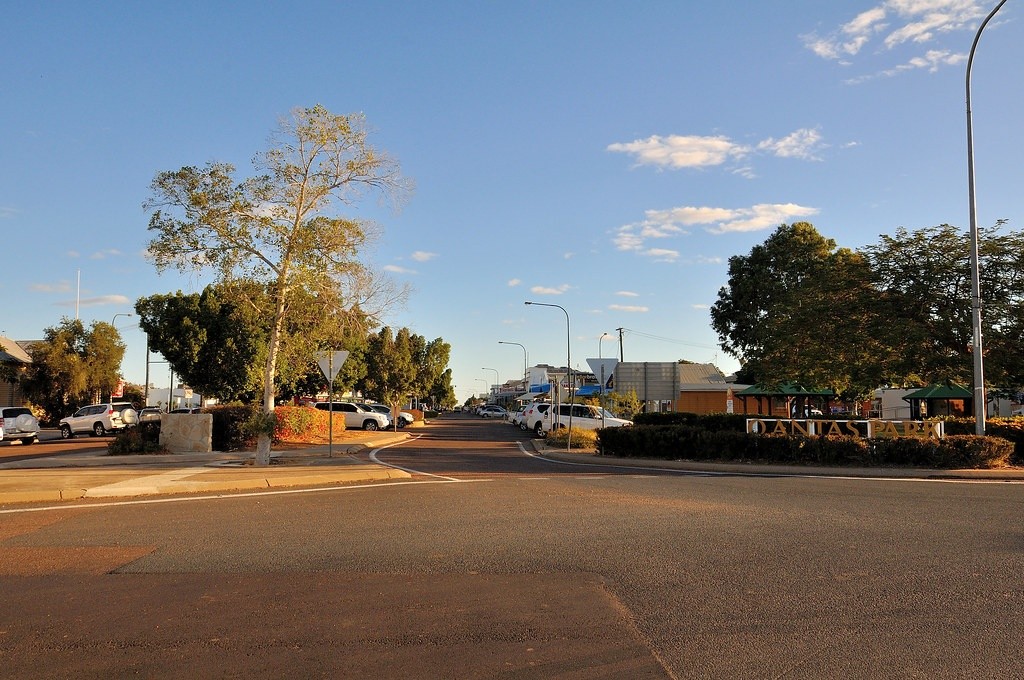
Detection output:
[525,301,571,404]
[499,342,527,391]
[475,368,498,399]
[599,333,607,358]
[109,314,132,403]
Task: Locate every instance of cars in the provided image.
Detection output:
[476,405,509,417]
[139,408,201,426]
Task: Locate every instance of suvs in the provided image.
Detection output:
[792,405,823,418]
[313,402,389,431]
[508,403,633,438]
[454,406,461,413]
[0,407,40,445]
[357,403,413,430]
[58,402,140,439]
